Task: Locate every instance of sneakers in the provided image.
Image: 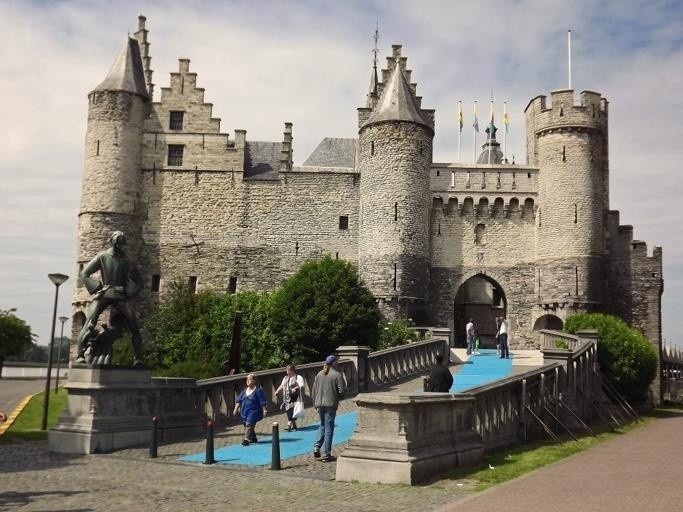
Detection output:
[242,440,250,445]
[284,425,298,432]
[249,438,258,444]
[323,455,336,462]
[467,351,480,356]
[496,350,509,359]
[313,448,321,458]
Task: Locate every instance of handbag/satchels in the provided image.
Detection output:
[288,374,300,402]
[292,394,306,420]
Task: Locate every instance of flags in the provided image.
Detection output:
[489,102,497,135]
[504,112,509,134]
[458,103,465,132]
[473,103,479,132]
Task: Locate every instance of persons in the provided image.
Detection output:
[426,355,453,392]
[494,317,501,357]
[499,316,510,359]
[75,229,146,367]
[311,355,345,464]
[466,319,474,355]
[473,322,481,355]
[275,363,305,432]
[232,372,269,445]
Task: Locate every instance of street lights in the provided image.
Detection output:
[40,273,70,430]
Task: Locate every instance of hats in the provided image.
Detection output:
[326,355,339,365]
